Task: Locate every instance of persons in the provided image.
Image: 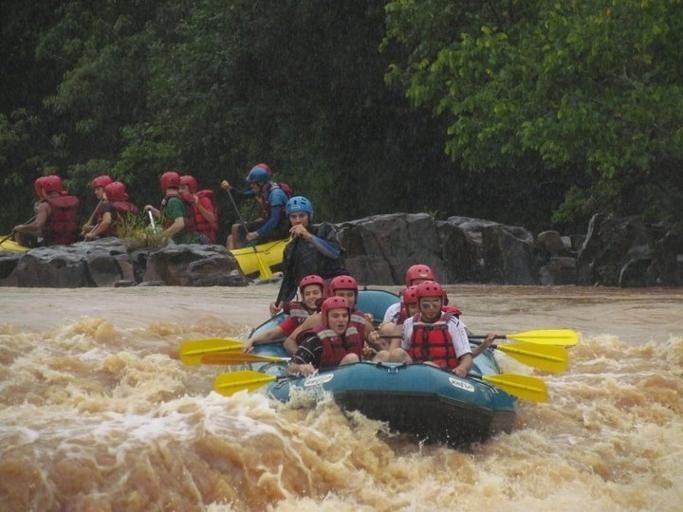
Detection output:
[179,176,219,244]
[242,265,496,379]
[32,177,49,217]
[13,174,78,250]
[143,171,201,246]
[226,163,294,251]
[221,166,290,250]
[80,174,115,239]
[269,196,349,316]
[85,181,139,242]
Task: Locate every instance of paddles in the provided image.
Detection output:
[179,337,287,364]
[469,340,568,372]
[199,354,292,363]
[227,188,273,280]
[447,370,547,401]
[214,370,301,397]
[380,330,578,345]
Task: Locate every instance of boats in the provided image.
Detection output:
[0,231,295,281]
[247,289,515,446]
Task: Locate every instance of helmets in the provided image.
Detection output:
[160,171,181,192]
[42,175,62,195]
[104,181,125,202]
[246,166,268,183]
[403,264,443,306]
[299,274,325,292]
[285,195,314,221]
[180,174,198,191]
[321,296,351,317]
[35,176,44,195]
[329,275,358,296]
[92,175,113,190]
[257,163,271,175]
